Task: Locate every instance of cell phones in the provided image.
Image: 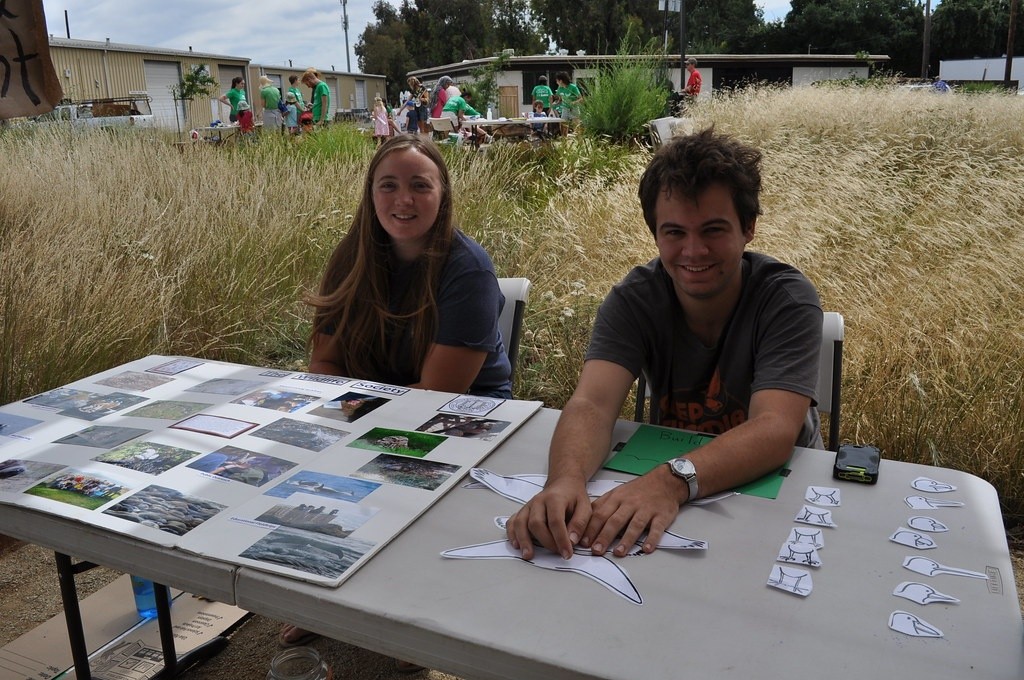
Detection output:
[833,444,881,484]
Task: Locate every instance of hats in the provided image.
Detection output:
[407,101,414,106]
[286,92,297,102]
[684,58,697,65]
[237,101,250,111]
[258,76,275,88]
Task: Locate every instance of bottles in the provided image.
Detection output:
[265,647,331,680]
[487,107,492,120]
[129,574,173,619]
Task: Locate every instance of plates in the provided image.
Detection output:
[512,118,526,120]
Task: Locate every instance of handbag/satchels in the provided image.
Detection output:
[278,99,289,116]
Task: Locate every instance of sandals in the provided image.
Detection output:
[395,659,425,674]
[278,623,319,649]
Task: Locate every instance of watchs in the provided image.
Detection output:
[664,457,699,501]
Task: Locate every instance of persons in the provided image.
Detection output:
[279,136,512,673]
[188,450,252,474]
[54,474,122,500]
[260,68,330,134]
[933,76,947,93]
[241,390,320,413]
[371,76,486,144]
[219,77,255,138]
[529,72,584,141]
[683,57,701,106]
[506,127,824,560]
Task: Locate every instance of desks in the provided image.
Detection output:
[462,118,565,149]
[0,355,1022,680]
[197,122,264,144]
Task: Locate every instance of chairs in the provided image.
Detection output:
[494,276,534,370]
[427,115,457,142]
[633,309,849,449]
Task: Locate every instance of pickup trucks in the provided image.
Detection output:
[32,90,158,130]
